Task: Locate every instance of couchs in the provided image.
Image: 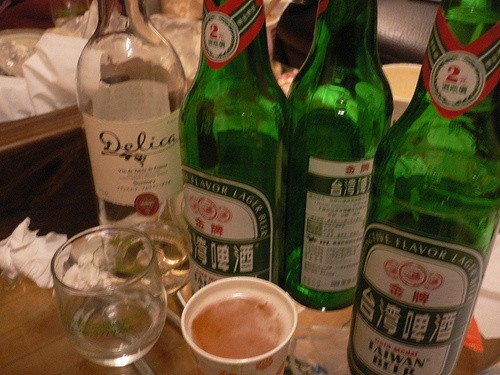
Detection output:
[273,0,444,75]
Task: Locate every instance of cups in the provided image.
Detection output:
[182,276,297,374]
[381,63,422,122]
[51,228,167,375]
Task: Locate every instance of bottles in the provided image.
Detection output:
[288,0,395,312]
[347,0,500,375]
[178,0,288,311]
[76,0,189,296]
